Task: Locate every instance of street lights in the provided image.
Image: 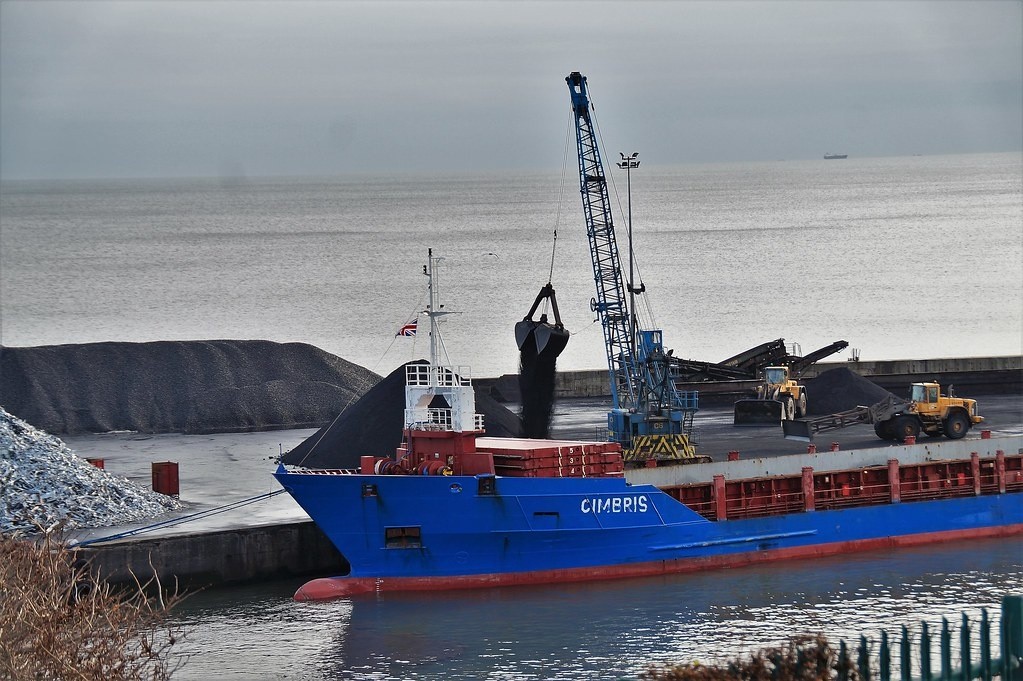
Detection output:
[615,152,641,357]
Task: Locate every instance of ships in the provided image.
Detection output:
[274,242,1023,600]
[824,152,847,159]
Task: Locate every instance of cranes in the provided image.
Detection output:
[513,71,715,468]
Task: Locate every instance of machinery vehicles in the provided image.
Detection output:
[733,366,808,426]
[780,382,984,444]
[618,337,859,397]
